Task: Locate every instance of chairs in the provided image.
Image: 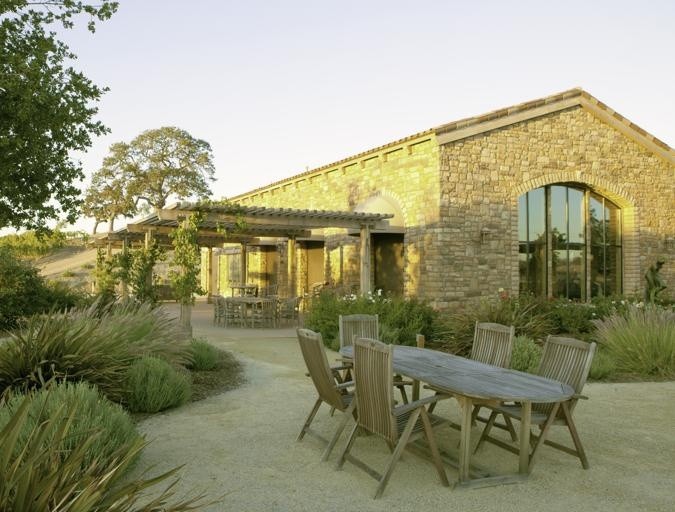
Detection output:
[423,319,516,449]
[334,336,453,499]
[298,327,398,462]
[213,284,303,327]
[338,313,379,378]
[472,334,598,477]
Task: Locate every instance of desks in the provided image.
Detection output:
[339,344,575,493]
